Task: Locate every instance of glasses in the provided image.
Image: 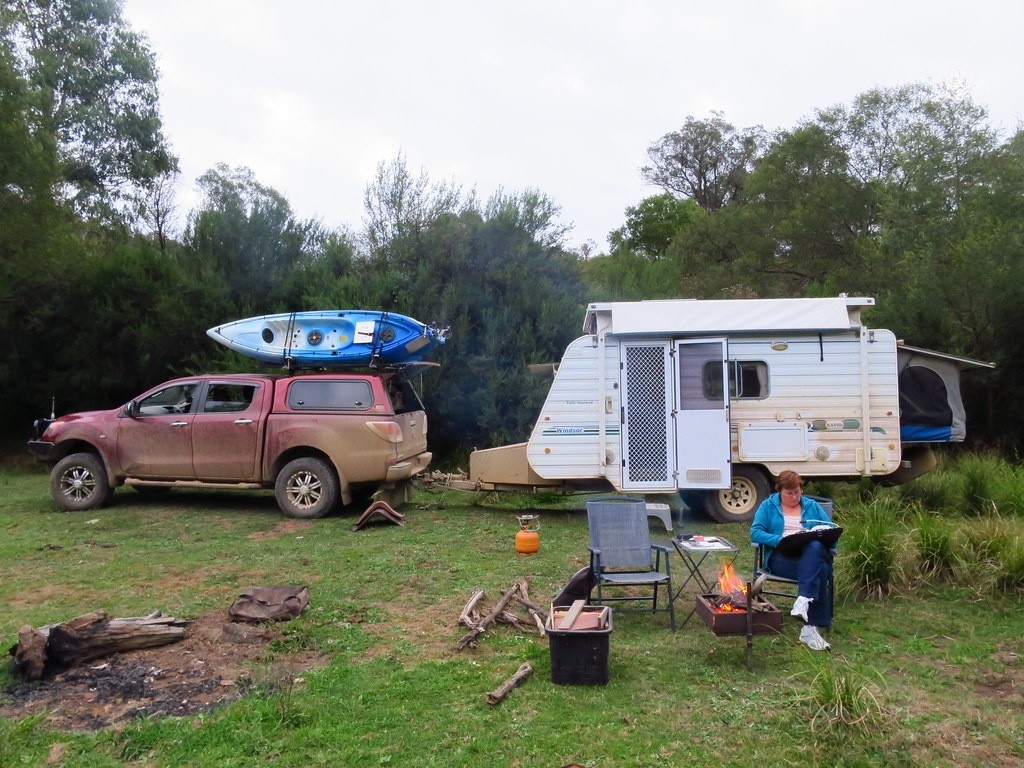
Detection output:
[780,488,803,497]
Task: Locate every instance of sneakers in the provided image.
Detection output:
[791,596,832,651]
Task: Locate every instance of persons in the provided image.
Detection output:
[749,471,833,650]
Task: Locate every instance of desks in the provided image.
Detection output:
[664,535,741,630]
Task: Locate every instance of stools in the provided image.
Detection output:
[645,503,673,531]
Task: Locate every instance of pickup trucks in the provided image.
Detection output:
[29,368,434,518]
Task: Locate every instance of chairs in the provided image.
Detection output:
[585,496,676,633]
[751,495,838,633]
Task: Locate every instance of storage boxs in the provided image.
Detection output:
[544,605,613,686]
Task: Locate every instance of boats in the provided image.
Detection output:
[205,310,451,371]
